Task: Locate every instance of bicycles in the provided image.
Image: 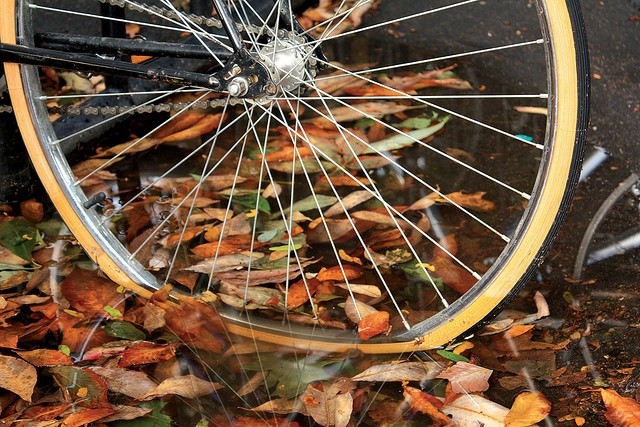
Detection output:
[0,0,592,356]
